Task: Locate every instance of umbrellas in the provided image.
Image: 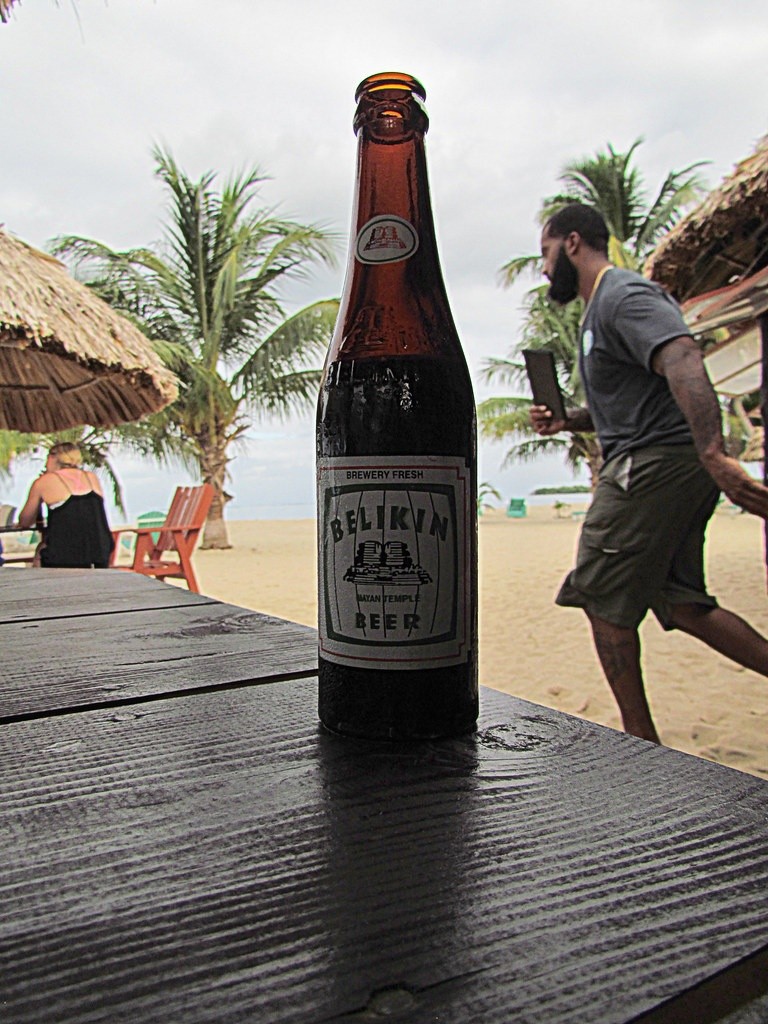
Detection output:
[0,228,179,434]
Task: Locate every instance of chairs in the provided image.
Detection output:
[506,498,527,518]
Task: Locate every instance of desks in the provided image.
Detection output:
[0,564,768,1024]
[0,523,49,567]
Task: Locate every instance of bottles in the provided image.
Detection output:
[315,72,478,738]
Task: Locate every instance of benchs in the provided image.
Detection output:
[111,484,217,594]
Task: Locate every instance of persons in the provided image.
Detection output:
[17,440,115,567]
[527,204,768,752]
